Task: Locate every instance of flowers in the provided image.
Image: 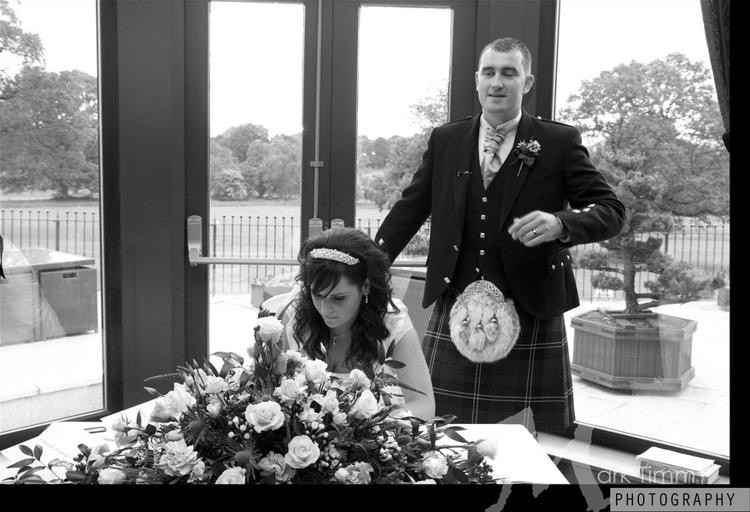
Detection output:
[8,313,499,485]
[513,138,541,180]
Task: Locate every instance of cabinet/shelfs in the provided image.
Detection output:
[1,244,100,349]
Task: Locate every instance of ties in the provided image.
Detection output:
[479,127,505,191]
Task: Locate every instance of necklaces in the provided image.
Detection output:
[329,332,352,349]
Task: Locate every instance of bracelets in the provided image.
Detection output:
[557,215,564,230]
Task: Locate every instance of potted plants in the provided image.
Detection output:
[543,43,733,395]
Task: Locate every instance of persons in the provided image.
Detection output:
[375,37,625,433]
[259,228,436,423]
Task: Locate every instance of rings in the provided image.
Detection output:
[532,229,538,236]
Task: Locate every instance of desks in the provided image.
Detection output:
[0,413,573,485]
